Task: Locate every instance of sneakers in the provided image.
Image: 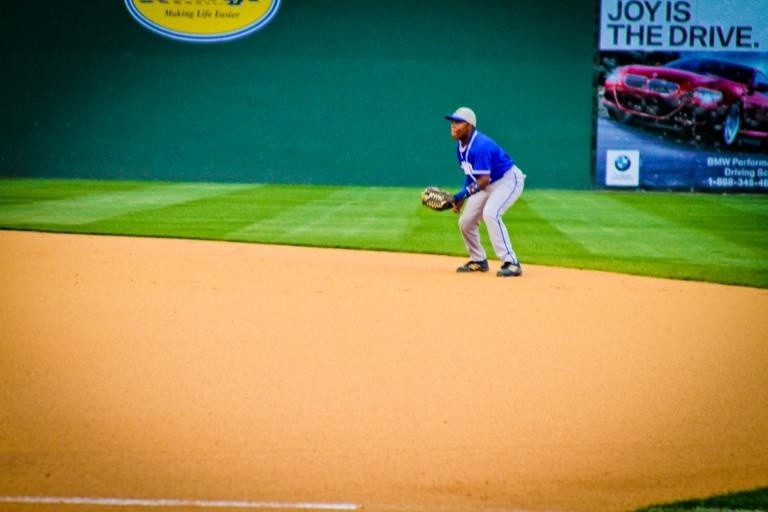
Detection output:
[456,258,489,272]
[496,261,522,276]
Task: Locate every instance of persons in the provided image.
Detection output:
[422,106,525,277]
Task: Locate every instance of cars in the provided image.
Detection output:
[602,55,768,148]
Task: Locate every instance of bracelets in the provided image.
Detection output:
[454,182,479,204]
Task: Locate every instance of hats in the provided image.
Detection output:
[445,106,477,128]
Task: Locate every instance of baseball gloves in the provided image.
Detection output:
[422,187,454,211]
